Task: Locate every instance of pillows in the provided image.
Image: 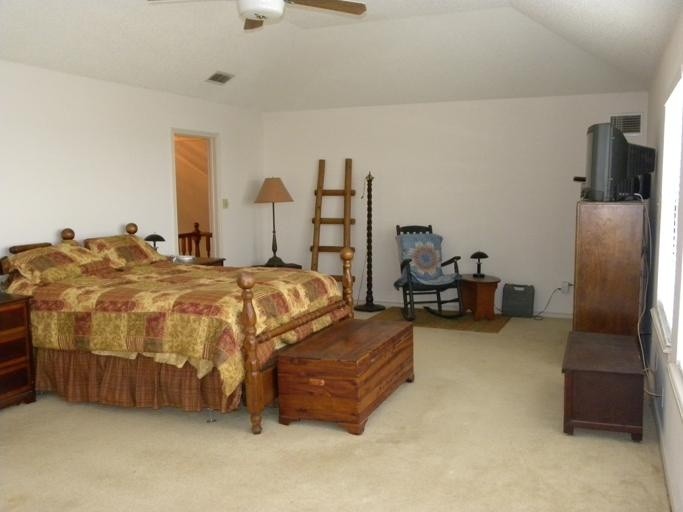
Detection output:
[82,234,168,273]
[395,233,444,280]
[4,241,122,292]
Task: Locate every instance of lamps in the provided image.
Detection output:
[254,177,294,267]
[144,234,166,255]
[235,0,285,20]
[469,251,488,279]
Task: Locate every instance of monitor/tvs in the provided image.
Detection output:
[583,122,655,202]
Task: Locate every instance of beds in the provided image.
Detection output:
[0,221,356,436]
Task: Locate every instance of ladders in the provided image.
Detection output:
[310,158,355,300]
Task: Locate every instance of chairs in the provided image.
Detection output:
[391,224,468,324]
[176,222,212,258]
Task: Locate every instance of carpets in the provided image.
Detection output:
[363,305,513,335]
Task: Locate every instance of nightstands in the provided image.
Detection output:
[173,255,227,266]
[0,290,36,412]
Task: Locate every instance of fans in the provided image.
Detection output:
[146,0,367,31]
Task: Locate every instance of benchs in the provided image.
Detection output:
[270,314,416,438]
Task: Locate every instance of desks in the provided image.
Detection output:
[453,272,502,321]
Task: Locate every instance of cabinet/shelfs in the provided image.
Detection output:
[561,330,645,444]
[572,199,650,331]
